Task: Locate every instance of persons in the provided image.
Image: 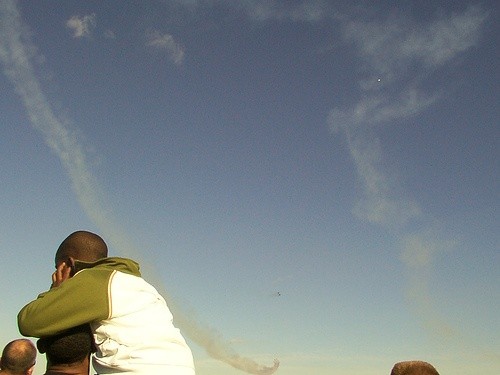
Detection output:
[0,322,96,375]
[17,230,196,375]
[390,360,440,375]
